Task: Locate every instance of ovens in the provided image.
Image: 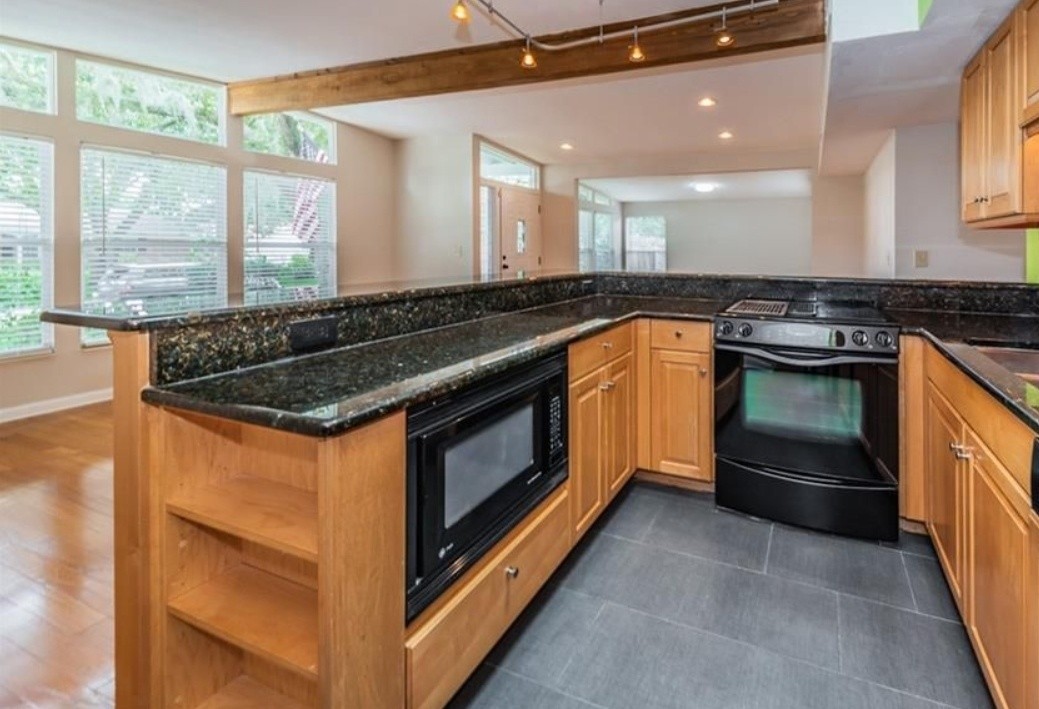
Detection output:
[711,341,901,549]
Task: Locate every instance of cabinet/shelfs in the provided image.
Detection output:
[154,316,711,709]
[904,346,1039,709]
[956,0,1039,230]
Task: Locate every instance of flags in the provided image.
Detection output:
[293,131,334,241]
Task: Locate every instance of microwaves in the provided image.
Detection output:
[405,345,569,628]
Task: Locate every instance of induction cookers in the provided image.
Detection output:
[718,285,901,353]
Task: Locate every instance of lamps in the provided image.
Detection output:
[629,26,645,63]
[448,0,474,25]
[715,9,736,47]
[520,37,539,71]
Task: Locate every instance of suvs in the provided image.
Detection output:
[98,262,190,297]
